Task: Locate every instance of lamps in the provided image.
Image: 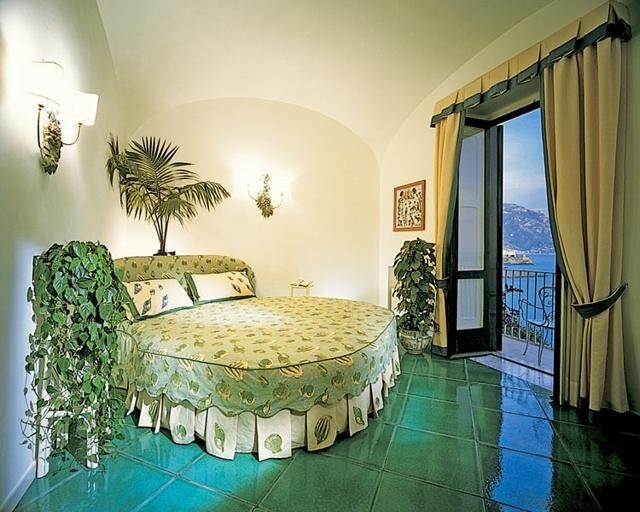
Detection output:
[246,170,284,218]
[23,59,99,175]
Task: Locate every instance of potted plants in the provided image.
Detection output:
[392,237,439,355]
[37,235,135,478]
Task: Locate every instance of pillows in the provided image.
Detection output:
[121,278,194,322]
[184,267,256,304]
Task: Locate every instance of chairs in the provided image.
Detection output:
[518,287,556,367]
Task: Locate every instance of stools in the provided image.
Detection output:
[290,285,311,297]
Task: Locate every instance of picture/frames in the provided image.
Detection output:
[391,180,427,232]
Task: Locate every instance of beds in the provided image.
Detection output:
[111,256,402,461]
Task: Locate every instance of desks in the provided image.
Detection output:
[35,325,101,479]
[388,266,399,311]
